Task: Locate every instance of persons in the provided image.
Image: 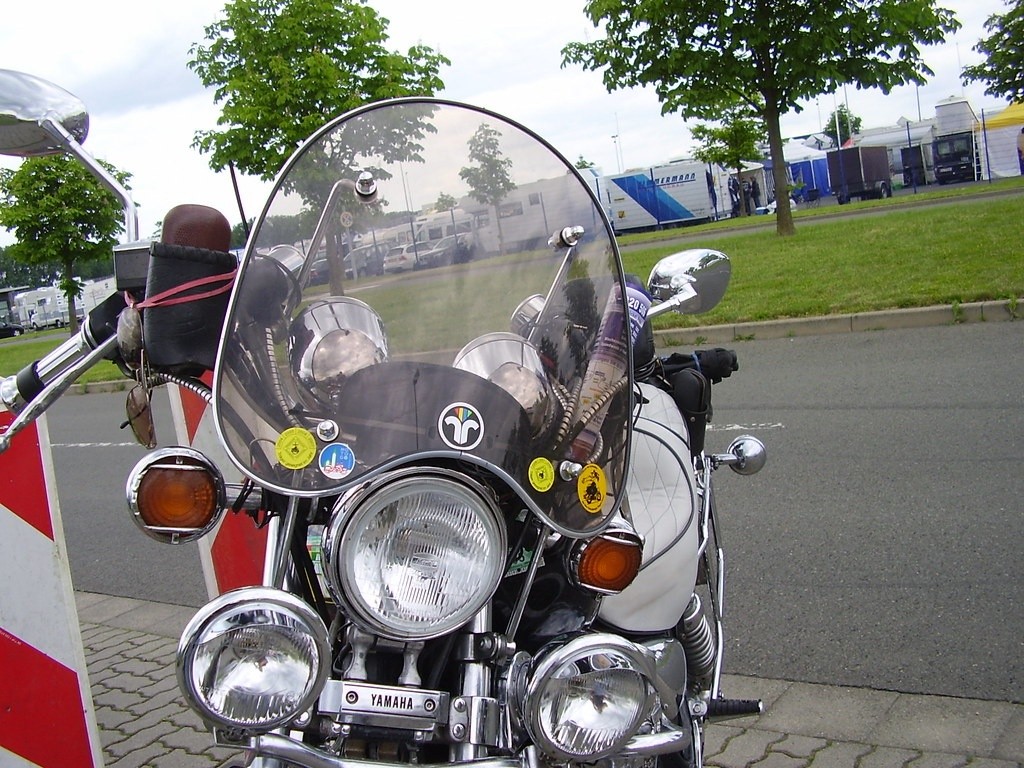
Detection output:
[742,176,761,216]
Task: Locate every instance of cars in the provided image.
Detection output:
[310,258,333,285]
[0,322,24,339]
[383,242,433,276]
[418,231,471,266]
[342,241,396,280]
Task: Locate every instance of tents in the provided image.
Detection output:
[974,101,1024,180]
[762,138,832,205]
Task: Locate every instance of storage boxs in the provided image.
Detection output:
[755,207,768,215]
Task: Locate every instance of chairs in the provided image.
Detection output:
[805,189,819,209]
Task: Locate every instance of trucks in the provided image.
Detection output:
[31,293,82,330]
[931,128,980,184]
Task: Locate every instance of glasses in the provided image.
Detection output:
[117,301,157,450]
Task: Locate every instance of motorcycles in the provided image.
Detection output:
[0,69,767,767]
